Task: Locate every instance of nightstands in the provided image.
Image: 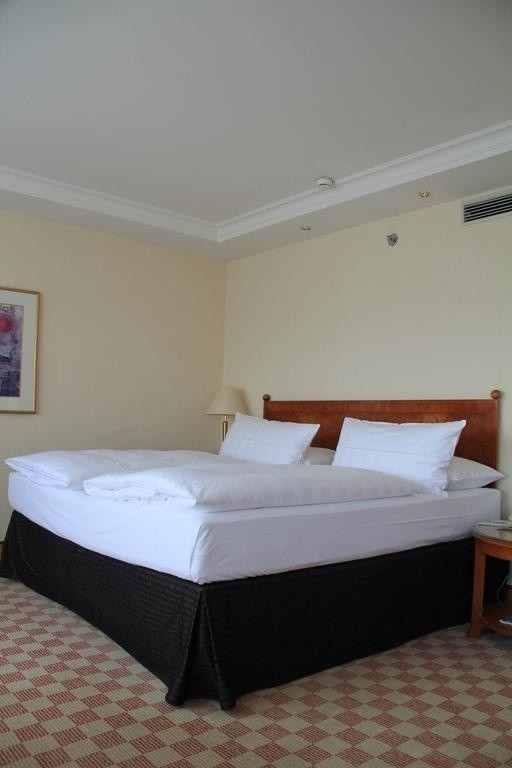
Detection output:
[467,525,512,639]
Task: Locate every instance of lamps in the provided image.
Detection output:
[204,385,246,442]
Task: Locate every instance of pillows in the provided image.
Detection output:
[217,411,321,465]
[333,417,466,496]
[305,447,336,467]
[445,455,507,492]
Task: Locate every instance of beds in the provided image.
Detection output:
[0,388,509,711]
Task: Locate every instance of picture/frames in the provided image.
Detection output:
[0,286,41,414]
